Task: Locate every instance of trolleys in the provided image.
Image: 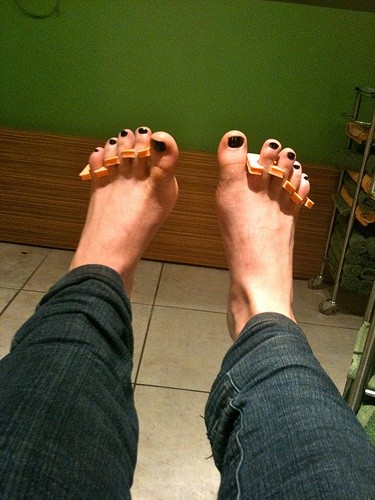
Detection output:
[308,84,375,318]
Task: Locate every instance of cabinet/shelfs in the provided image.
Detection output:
[308,84,375,317]
[343,281,375,418]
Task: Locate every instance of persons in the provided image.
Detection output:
[0,126,375,500]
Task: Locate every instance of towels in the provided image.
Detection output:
[346,319,375,449]
[322,83,375,298]
